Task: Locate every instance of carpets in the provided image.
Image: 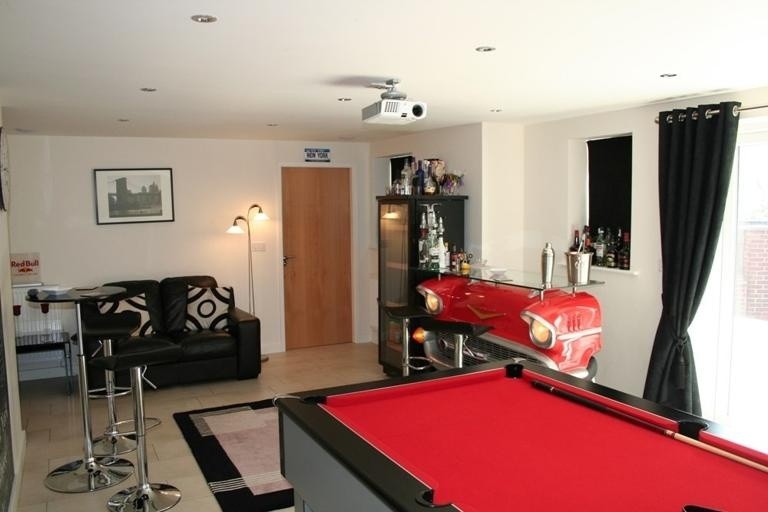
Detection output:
[171,398,296,511]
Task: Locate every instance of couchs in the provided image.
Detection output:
[78,274,261,400]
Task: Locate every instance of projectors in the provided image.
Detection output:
[361,98,428,126]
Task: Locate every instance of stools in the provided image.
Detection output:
[71,310,182,511]
[419,319,495,371]
[377,300,435,378]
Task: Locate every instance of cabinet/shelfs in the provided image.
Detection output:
[376,195,468,377]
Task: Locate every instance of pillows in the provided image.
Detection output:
[96,292,156,338]
[183,283,232,332]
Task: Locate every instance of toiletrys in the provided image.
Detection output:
[541,243,555,285]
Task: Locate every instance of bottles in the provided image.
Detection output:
[418,212,471,275]
[392,158,441,197]
[569,225,630,271]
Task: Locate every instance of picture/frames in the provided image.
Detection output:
[93,167,174,225]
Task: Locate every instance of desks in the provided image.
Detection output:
[23,285,135,494]
[413,258,606,381]
[275,358,768,512]
[15,331,74,395]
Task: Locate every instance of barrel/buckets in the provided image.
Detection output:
[565,239,594,286]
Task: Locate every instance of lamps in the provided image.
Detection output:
[225,202,272,363]
